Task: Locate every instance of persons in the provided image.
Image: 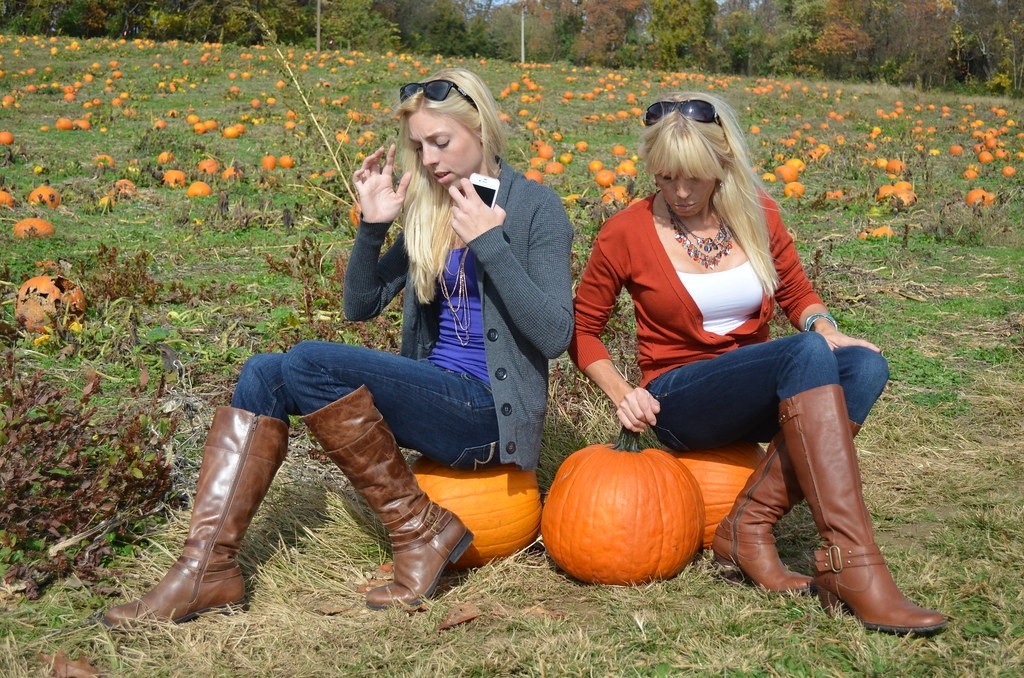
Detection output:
[573,91,944,639]
[94,66,576,635]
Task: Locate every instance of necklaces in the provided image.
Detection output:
[439,246,470,346]
[663,198,733,270]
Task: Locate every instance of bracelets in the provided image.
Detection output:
[804,313,838,332]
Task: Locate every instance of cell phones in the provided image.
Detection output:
[465,172,500,210]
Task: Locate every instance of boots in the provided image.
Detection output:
[104,406,288,635]
[712,415,862,595]
[779,383,946,633]
[301,383,473,612]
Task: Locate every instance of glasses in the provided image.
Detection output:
[400,79,479,113]
[641,99,723,128]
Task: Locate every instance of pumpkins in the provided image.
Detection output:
[542,424,704,585]
[0,35,1024,239]
[658,442,768,551]
[406,455,542,566]
